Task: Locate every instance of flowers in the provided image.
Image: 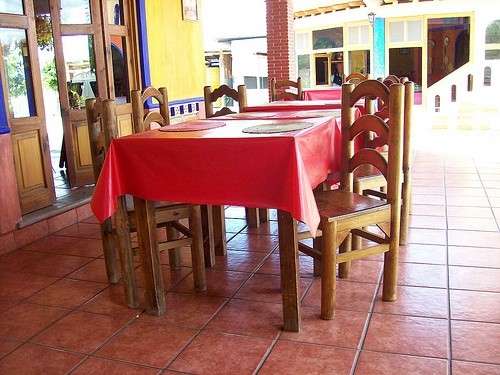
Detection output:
[36,17,54,51]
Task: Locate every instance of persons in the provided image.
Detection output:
[331,68,343,87]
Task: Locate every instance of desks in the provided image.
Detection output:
[244,100,365,115]
[303,88,342,99]
[91,109,360,332]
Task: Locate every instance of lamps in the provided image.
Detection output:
[368,12,376,24]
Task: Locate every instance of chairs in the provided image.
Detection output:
[86,72,413,321]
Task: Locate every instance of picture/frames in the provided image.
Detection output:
[182,0,199,22]
